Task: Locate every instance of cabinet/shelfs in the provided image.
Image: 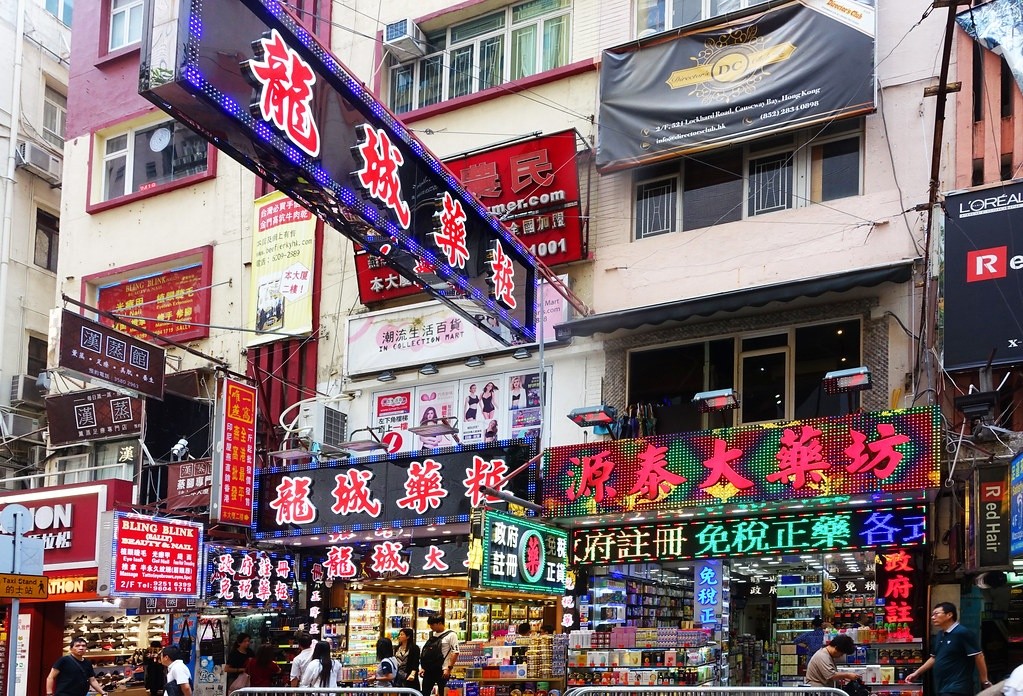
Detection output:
[62,621,166,656]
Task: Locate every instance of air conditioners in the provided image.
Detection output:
[4,413,39,441]
[29,472,44,487]
[10,374,47,408]
[382,16,426,63]
[19,142,50,176]
[299,404,347,456]
[31,446,45,468]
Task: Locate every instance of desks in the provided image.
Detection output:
[464,675,566,696]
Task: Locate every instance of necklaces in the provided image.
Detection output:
[239,648,248,654]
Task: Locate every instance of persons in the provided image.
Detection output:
[519,623,553,636]
[143,641,169,696]
[465,382,500,444]
[394,629,420,696]
[46,638,109,696]
[368,638,399,696]
[905,601,993,696]
[420,407,442,451]
[299,641,343,696]
[420,614,460,696]
[290,633,317,696]
[473,313,501,349]
[510,377,526,409]
[160,646,194,696]
[795,615,824,674]
[516,411,524,425]
[245,645,282,696]
[853,614,867,628]
[224,633,254,689]
[806,635,862,696]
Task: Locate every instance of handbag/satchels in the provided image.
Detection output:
[212,620,225,664]
[200,620,216,655]
[178,620,192,664]
[228,672,251,695]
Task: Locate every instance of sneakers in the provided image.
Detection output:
[62,616,166,692]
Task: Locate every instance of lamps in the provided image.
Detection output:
[407,416,462,445]
[419,364,439,375]
[953,390,1002,419]
[464,356,485,367]
[338,426,390,454]
[267,435,319,462]
[567,404,618,440]
[824,367,872,414]
[690,388,741,428]
[512,348,533,359]
[377,370,397,382]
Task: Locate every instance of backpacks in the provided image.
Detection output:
[387,658,407,687]
[421,630,457,673]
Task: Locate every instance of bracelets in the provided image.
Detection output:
[47,693,53,695]
[102,692,108,696]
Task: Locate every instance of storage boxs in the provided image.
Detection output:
[452,626,730,696]
[866,665,881,683]
[856,647,866,663]
[846,650,856,663]
[880,667,894,684]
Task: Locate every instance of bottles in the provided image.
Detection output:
[658,666,714,685]
[823,622,907,645]
[763,598,824,650]
[767,652,778,687]
[832,594,876,608]
[823,609,875,625]
